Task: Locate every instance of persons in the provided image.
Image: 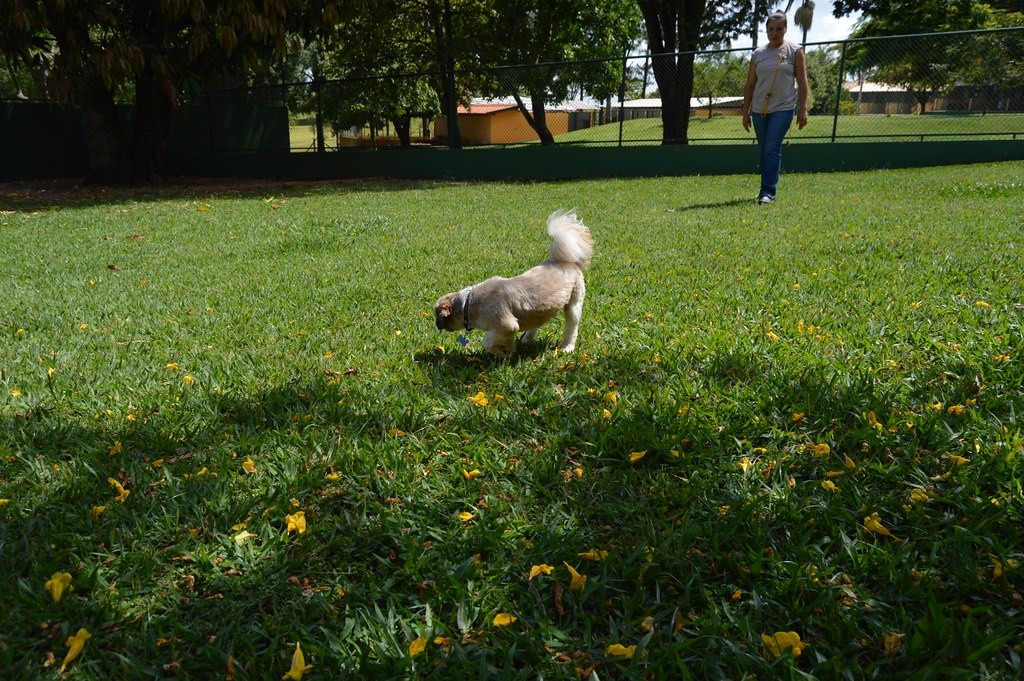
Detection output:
[742,9,808,203]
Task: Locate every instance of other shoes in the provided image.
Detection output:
[758,196,774,205]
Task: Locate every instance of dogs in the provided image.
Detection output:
[434,206,595,358]
